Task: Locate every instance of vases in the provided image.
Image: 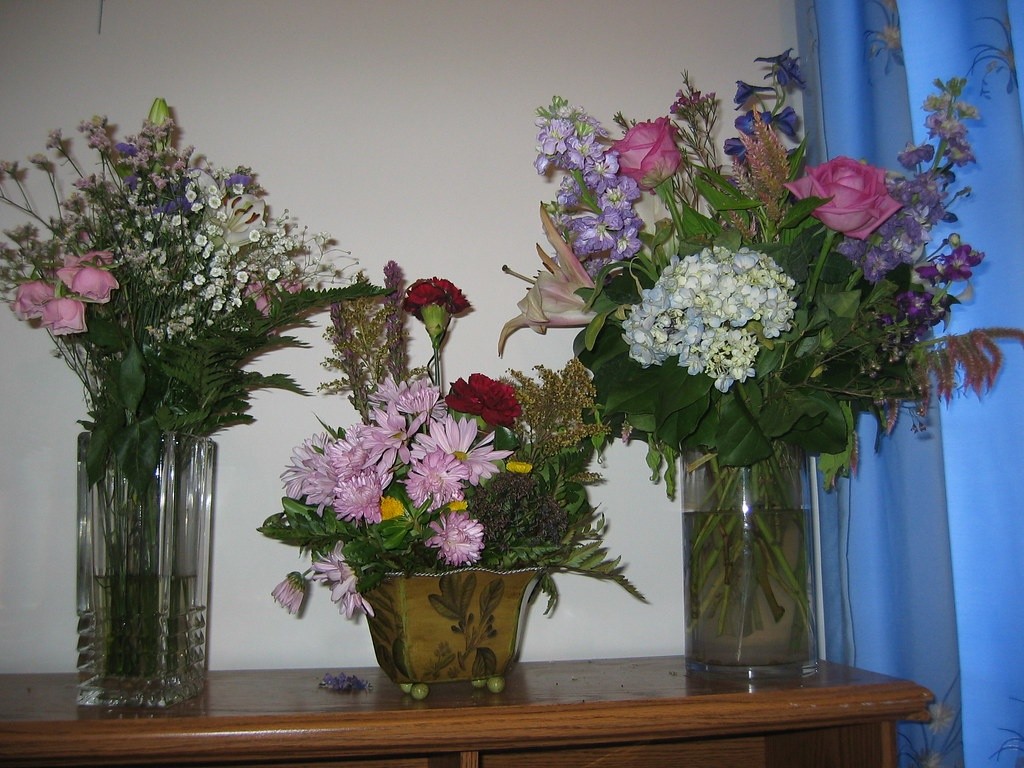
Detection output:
[360,567,541,698]
[76,430,219,707]
[682,440,818,681]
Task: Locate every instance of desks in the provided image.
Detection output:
[1,656,935,768]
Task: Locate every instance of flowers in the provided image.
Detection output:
[0,48,1024,619]
